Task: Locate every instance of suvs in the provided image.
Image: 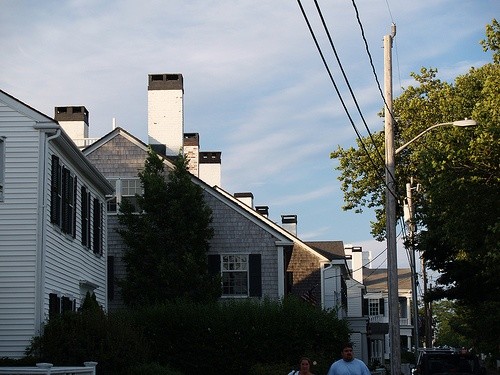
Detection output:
[409,348,473,374]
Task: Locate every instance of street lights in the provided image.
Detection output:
[383,34,477,375]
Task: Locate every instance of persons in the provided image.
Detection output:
[326,344,371,375]
[286,357,315,375]
